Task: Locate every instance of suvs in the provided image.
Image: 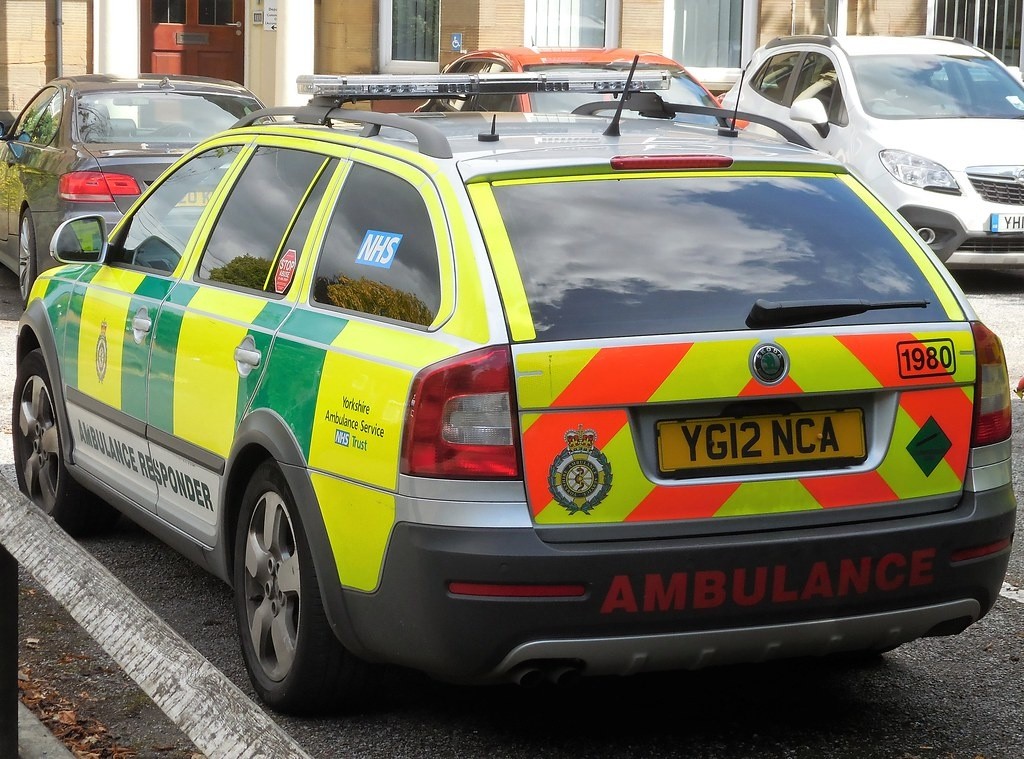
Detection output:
[717,34,1024,269]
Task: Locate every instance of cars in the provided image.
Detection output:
[1,75,276,309]
[411,48,753,131]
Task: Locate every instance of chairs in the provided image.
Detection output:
[110,118,137,137]
[80,103,114,137]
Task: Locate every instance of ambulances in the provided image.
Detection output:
[8,70,1018,719]
